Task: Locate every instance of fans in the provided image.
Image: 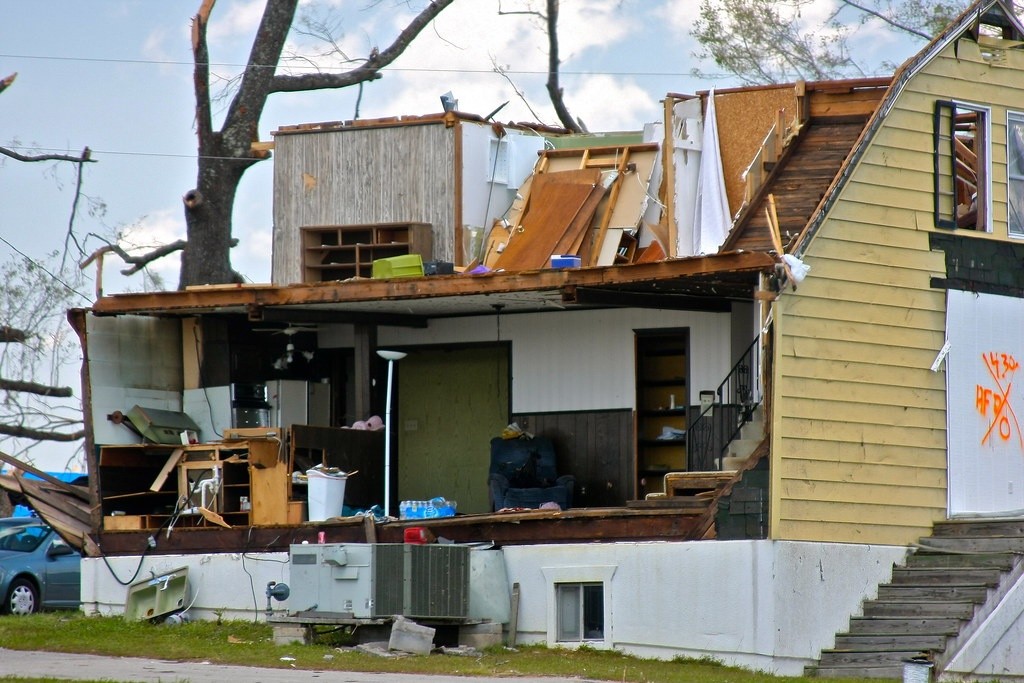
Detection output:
[253,321,327,336]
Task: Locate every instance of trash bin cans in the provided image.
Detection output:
[307,469,348,522]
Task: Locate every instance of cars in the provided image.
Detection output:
[0,517,81,617]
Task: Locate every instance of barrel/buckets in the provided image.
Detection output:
[371,254,425,279]
[306,470,347,522]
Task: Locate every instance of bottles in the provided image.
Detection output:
[400,496,457,520]
[165,612,189,624]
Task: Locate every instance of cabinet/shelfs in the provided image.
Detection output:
[246,426,394,525]
[99,443,182,530]
[298,223,434,281]
[175,445,250,527]
[642,350,688,476]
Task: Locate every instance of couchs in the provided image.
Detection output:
[488,435,582,510]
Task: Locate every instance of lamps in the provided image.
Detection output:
[274,345,313,368]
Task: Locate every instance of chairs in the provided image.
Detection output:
[20,535,37,546]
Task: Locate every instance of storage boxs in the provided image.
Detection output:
[550,254,581,269]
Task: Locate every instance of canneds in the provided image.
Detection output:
[317,532,325,544]
